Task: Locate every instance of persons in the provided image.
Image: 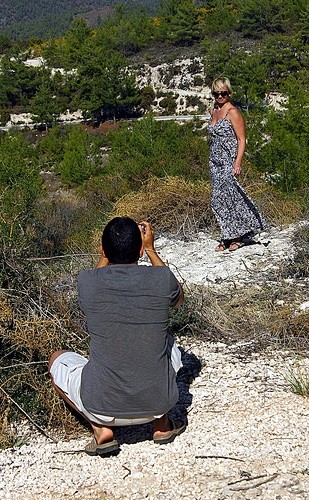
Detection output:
[48,217,186,456]
[207,76,268,252]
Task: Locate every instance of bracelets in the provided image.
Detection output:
[145,249,154,253]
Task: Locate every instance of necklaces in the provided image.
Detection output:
[218,103,227,111]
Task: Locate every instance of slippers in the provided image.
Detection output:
[215,242,228,251]
[228,241,246,251]
[85,437,120,456]
[152,414,186,443]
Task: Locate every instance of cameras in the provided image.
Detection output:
[136,223,145,234]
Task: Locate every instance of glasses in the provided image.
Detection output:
[211,91,230,97]
